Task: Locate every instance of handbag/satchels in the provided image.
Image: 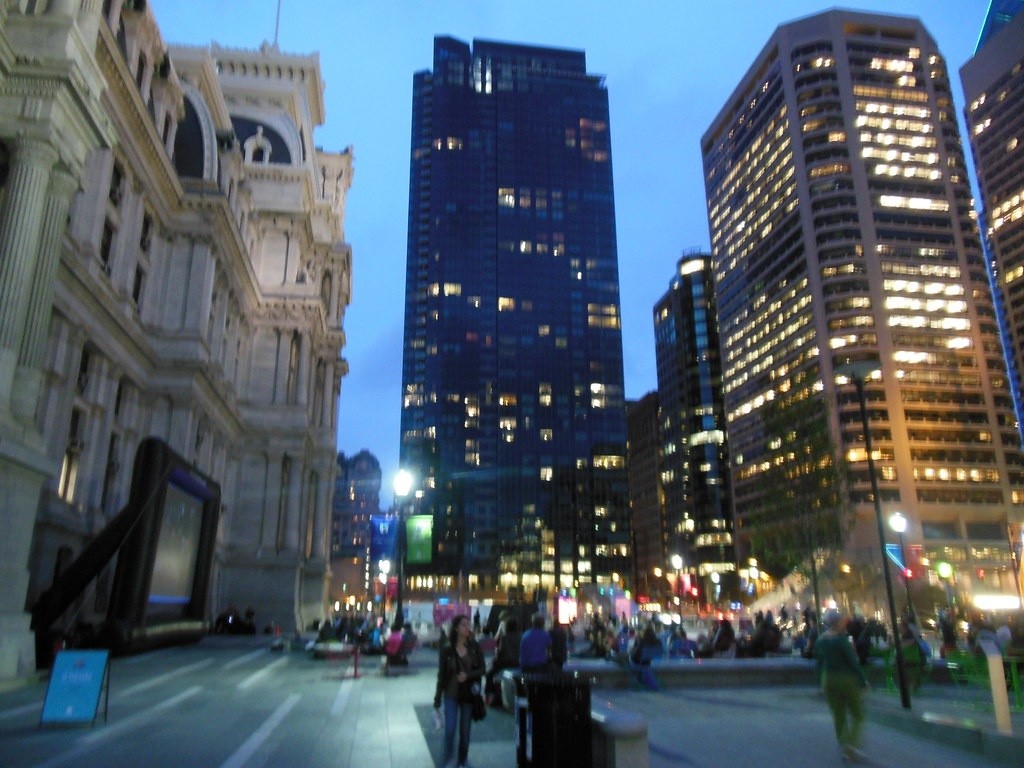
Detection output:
[471,692,486,722]
[917,639,933,658]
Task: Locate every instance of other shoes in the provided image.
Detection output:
[843,743,866,763]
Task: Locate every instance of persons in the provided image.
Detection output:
[815,611,874,760]
[292,578,982,688]
[432,614,487,768]
[519,613,554,674]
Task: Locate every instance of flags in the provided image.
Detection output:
[885,543,925,581]
